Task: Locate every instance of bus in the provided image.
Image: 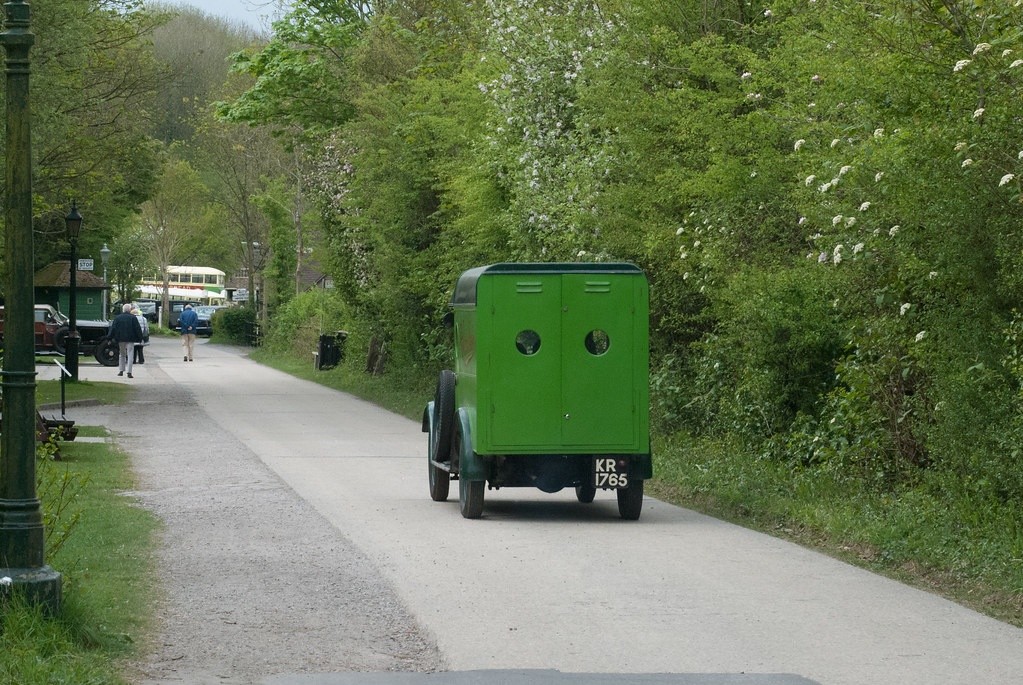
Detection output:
[112,266,228,301]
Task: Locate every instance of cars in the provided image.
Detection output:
[112,298,229,338]
[0,304,120,366]
[422,261,653,522]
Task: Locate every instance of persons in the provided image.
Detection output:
[180,304,198,361]
[106,303,149,378]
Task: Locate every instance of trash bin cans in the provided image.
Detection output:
[319,331,347,369]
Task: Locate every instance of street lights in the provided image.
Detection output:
[99,243,110,322]
[63,198,84,383]
